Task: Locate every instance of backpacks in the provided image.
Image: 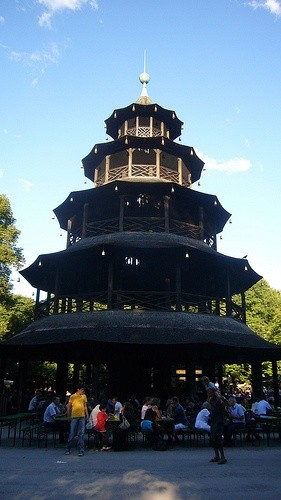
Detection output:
[244,410,256,427]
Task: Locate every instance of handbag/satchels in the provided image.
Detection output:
[152,438,166,450]
[119,415,130,429]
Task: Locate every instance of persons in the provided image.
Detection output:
[29,385,281,451]
[201,376,220,397]
[209,394,227,463]
[65,386,89,455]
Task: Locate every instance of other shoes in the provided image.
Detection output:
[66,452,69,455]
[217,458,227,464]
[209,457,220,462]
[103,446,111,450]
[78,452,84,455]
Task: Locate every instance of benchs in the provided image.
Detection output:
[82,431,89,450]
[38,428,48,447]
[176,428,193,449]
[127,432,136,450]
[233,429,250,447]
[143,431,151,449]
[21,418,38,448]
[1,423,11,441]
[194,430,207,448]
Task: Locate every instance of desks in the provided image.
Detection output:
[1,412,36,447]
[106,418,122,450]
[53,417,73,449]
[155,417,174,448]
[256,415,281,447]
